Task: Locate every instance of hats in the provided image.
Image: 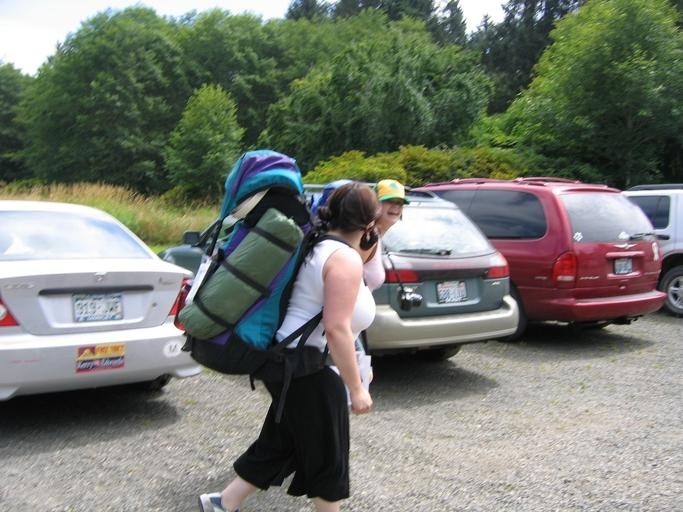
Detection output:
[372,179,410,205]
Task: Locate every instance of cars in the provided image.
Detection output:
[0,199,195,401]
[410,177,668,344]
[155,183,521,363]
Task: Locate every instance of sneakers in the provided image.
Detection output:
[199,491,241,512]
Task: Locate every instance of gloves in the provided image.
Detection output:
[311,179,351,214]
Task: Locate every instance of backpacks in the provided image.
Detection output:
[181,150,310,376]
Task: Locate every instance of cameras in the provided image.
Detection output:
[397,290,422,311]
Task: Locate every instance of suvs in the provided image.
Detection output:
[619,183,682,317]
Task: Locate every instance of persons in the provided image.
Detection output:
[196,181,383,511]
[310,177,423,406]
